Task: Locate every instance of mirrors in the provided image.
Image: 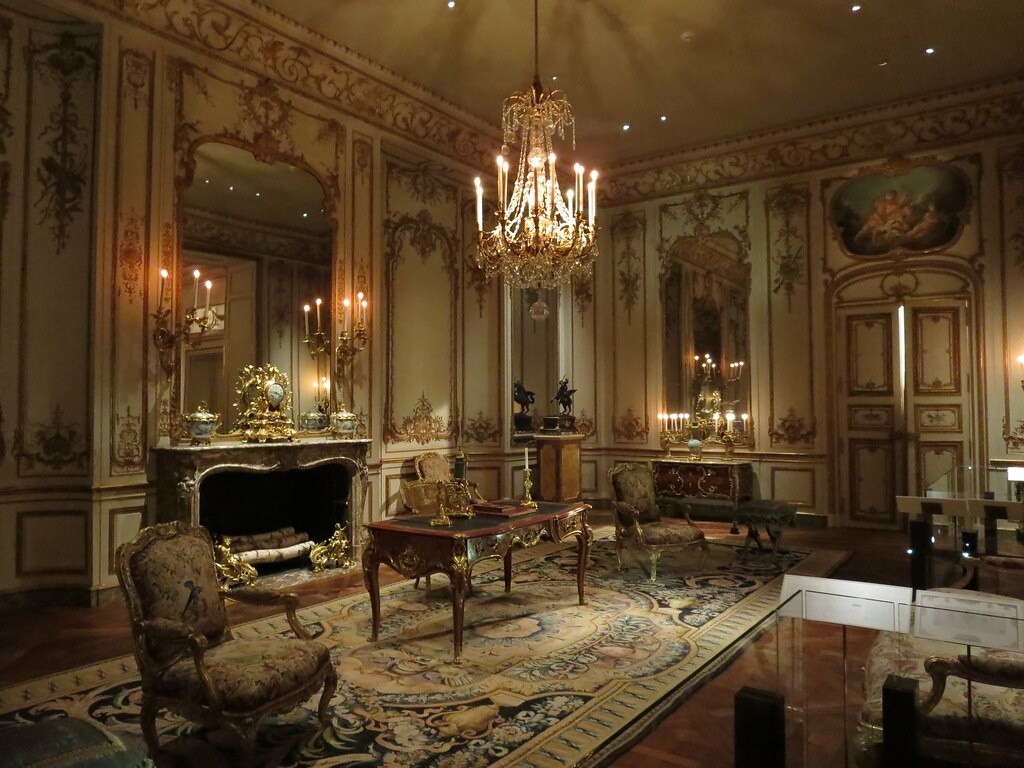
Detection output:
[658,229,755,447]
[176,132,341,439]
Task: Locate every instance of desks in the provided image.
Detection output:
[150,437,375,568]
[362,499,593,664]
[651,458,752,534]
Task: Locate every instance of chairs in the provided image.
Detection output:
[114,520,337,768]
[401,452,488,602]
[607,462,709,582]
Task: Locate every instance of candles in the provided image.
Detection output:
[303,305,310,335]
[659,412,735,432]
[160,269,168,310]
[316,299,321,327]
[661,414,748,433]
[357,292,363,318]
[205,280,212,316]
[193,269,200,308]
[343,299,350,330]
[1017,355,1024,379]
[362,301,367,323]
[474,152,597,233]
[523,447,530,470]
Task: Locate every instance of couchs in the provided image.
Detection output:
[856,586,1024,768]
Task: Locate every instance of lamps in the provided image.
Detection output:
[475,0,598,321]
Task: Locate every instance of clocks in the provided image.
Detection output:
[247,363,300,442]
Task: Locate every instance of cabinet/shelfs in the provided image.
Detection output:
[534,434,587,503]
